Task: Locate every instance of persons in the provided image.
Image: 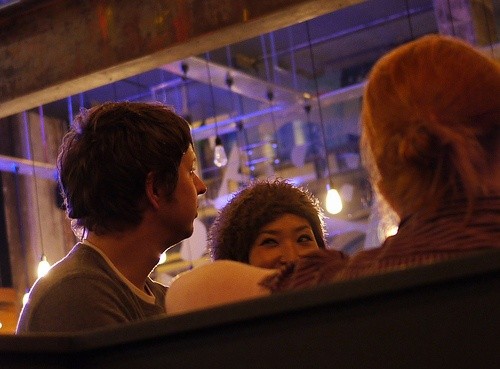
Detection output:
[13,99,209,334]
[207,176,328,270]
[259,34,500,294]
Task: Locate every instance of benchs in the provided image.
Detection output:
[0,254,500,369]
